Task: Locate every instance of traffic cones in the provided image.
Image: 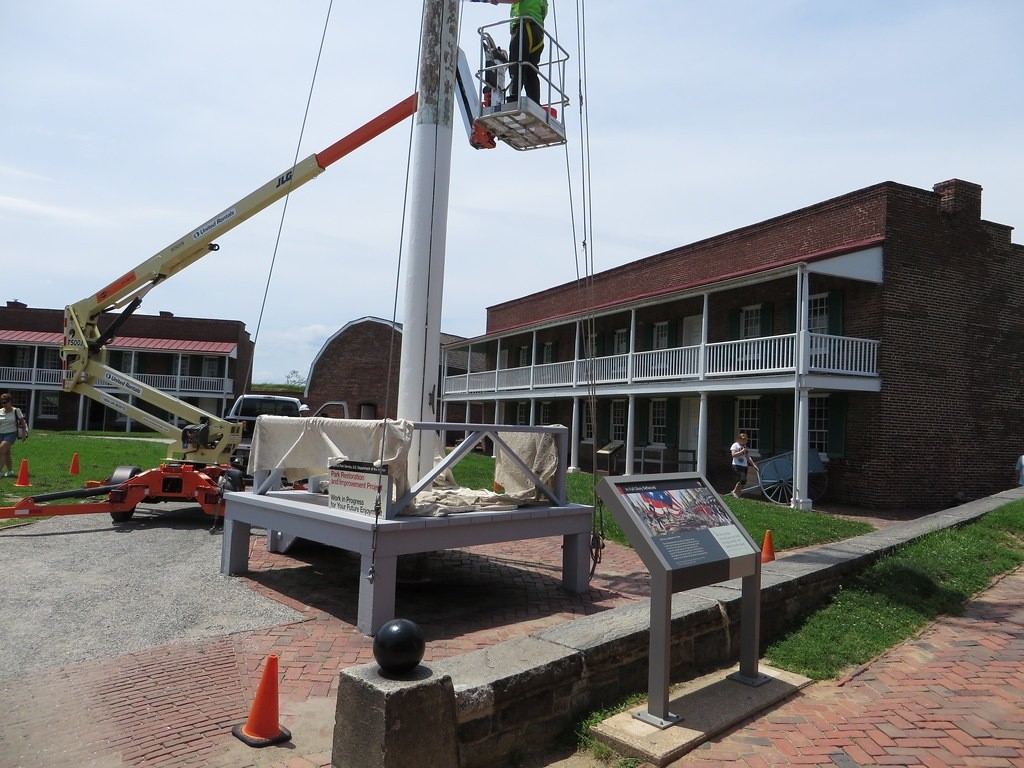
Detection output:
[232,654,292,748]
[68,452,79,475]
[13,458,34,487]
[761,530,775,564]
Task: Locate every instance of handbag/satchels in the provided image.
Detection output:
[15,408,28,439]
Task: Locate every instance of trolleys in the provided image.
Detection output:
[724,449,828,506]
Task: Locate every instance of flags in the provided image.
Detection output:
[641,490,673,510]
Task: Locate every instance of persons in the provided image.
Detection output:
[468,0,548,104]
[1015,454,1024,486]
[299,404,310,417]
[0,394,27,478]
[730,433,759,499]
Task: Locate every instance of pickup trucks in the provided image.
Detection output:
[223,395,311,481]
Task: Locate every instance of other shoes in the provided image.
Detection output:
[731,490,739,498]
[2,470,13,477]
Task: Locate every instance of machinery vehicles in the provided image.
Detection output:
[0,16,570,523]
